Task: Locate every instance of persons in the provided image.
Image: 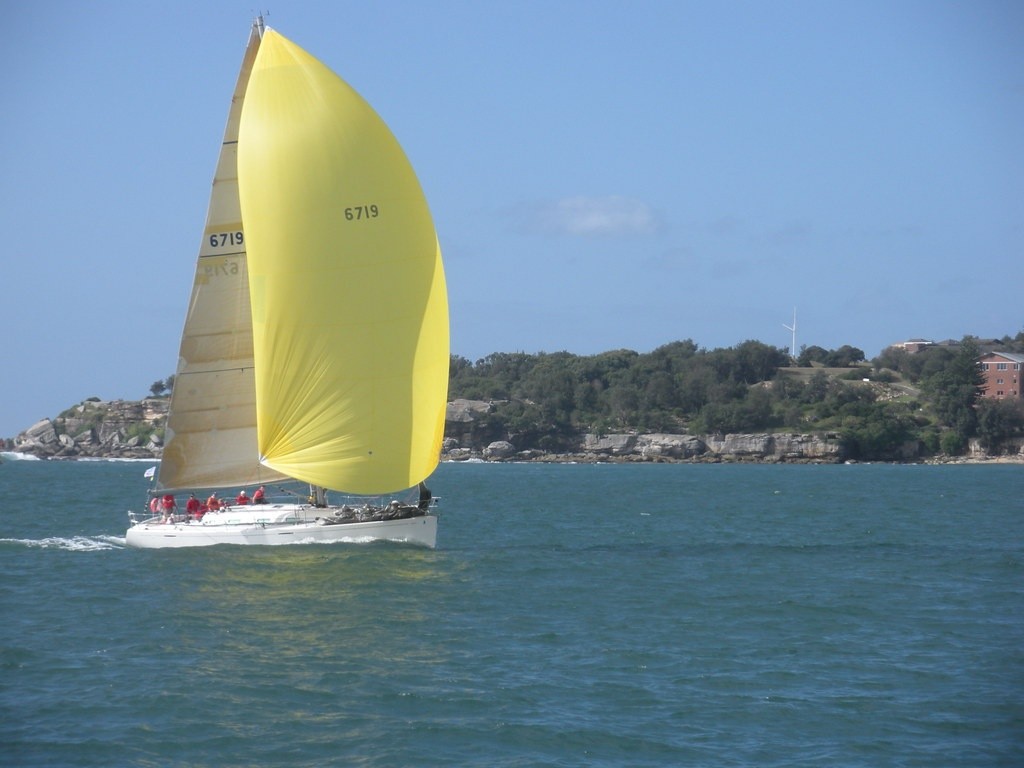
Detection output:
[161,495,178,524]
[253,484,265,505]
[186,492,230,522]
[236,491,252,505]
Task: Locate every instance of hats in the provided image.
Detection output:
[190,493,194,496]
[241,491,246,495]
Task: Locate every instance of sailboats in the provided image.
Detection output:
[123,5,451,549]
